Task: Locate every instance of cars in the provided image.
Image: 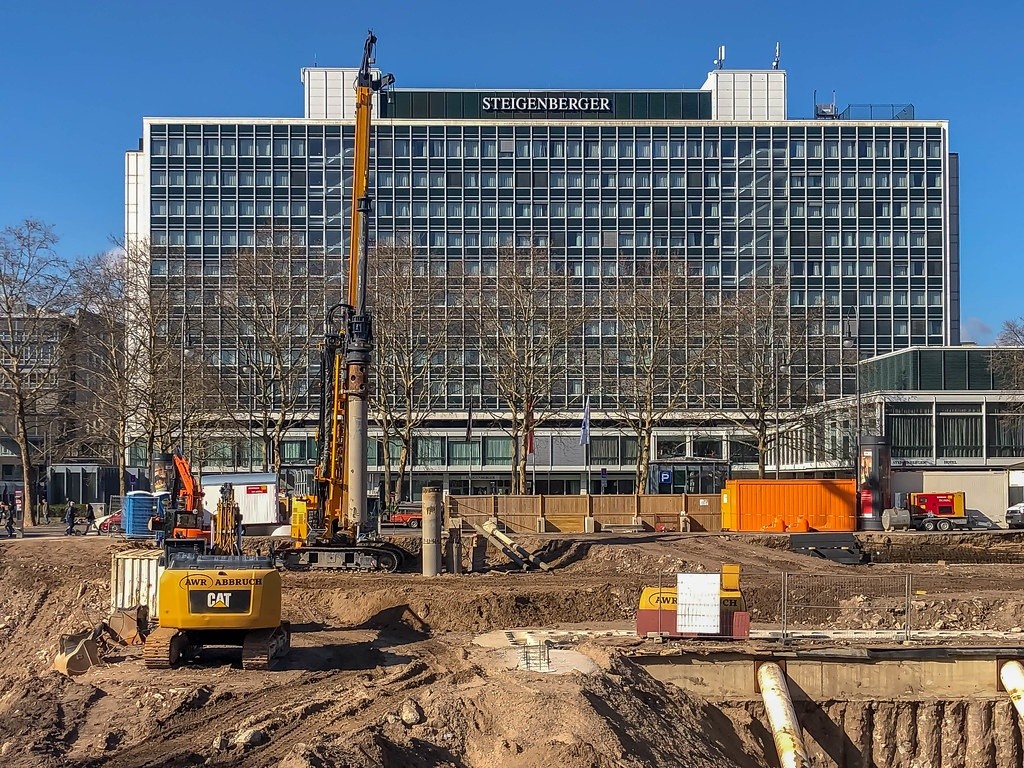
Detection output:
[91,509,122,534]
[1006,503,1024,529]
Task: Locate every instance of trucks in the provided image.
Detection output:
[387,513,423,528]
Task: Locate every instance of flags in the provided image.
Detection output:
[527,394,534,453]
[579,396,590,446]
[465,395,472,442]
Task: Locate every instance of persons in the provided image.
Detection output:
[82,504,101,536]
[0,499,49,538]
[64,501,75,535]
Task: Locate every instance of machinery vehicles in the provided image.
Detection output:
[144,482,291,670]
[271,32,409,575]
[147,451,206,549]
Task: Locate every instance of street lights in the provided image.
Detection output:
[775,338,788,480]
[842,305,861,532]
[239,353,252,474]
[180,312,195,456]
